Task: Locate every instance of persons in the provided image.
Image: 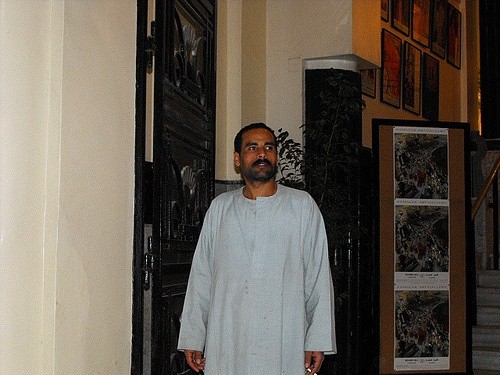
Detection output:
[177,123,337,375]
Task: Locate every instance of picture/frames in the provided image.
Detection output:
[372,118,473,375]
[359,0,462,120]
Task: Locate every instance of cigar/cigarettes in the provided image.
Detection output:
[307,368,317,375]
